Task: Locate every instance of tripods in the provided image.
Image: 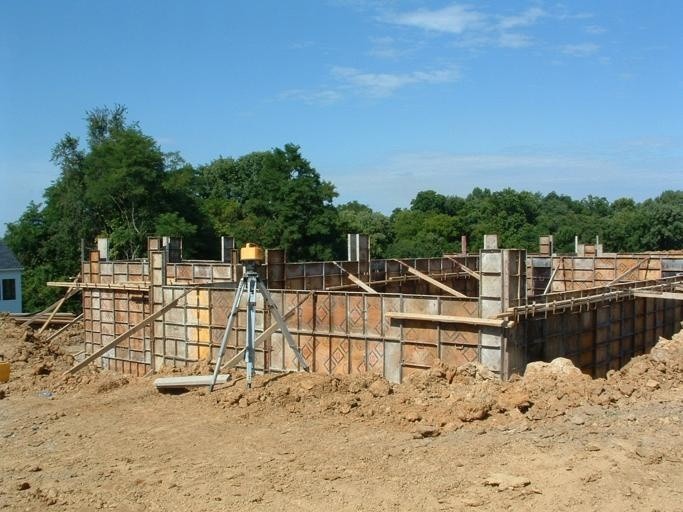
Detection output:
[209,271,310,393]
